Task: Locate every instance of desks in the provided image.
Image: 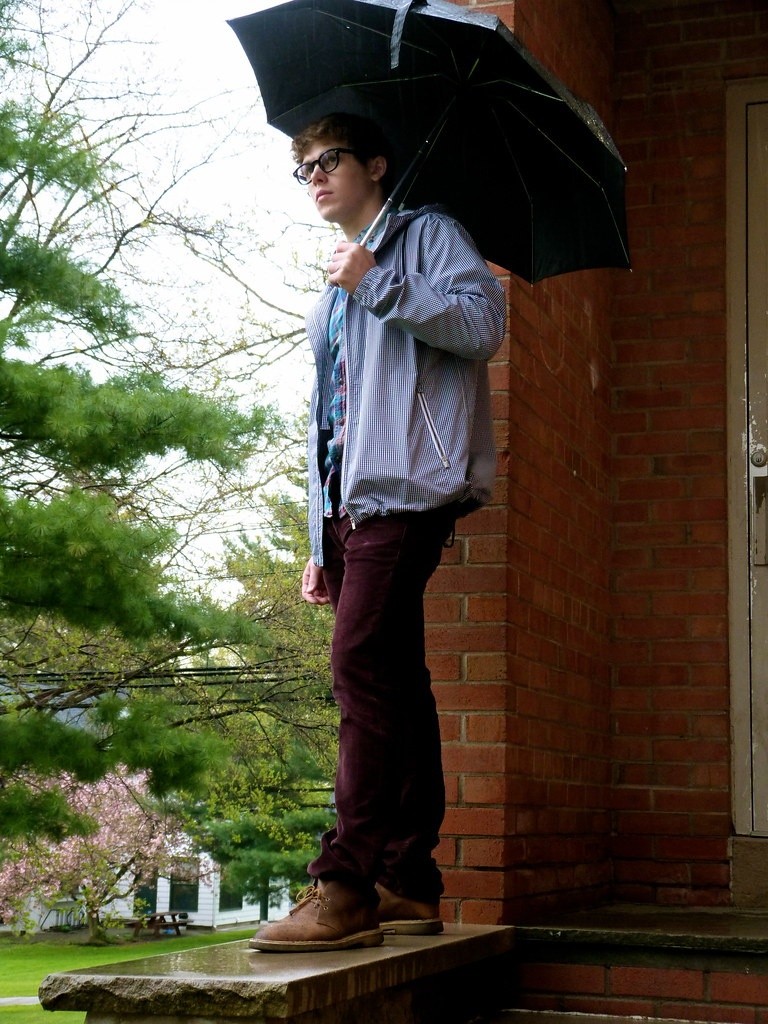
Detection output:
[133,912,181,938]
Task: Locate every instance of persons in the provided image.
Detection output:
[242,113,508,948]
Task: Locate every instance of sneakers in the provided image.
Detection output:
[249,878,384,952]
[374,878,444,934]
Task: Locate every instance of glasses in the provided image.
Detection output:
[293,147,375,185]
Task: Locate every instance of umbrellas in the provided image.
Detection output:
[229,0,640,290]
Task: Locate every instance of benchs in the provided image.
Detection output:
[124,921,156,929]
[156,922,187,929]
